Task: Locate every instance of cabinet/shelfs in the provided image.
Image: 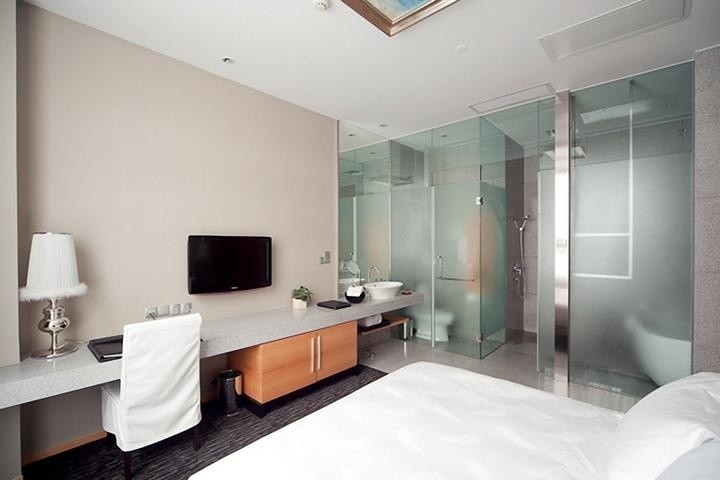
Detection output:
[0,292,425,480]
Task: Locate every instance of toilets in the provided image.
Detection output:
[404,283,456,343]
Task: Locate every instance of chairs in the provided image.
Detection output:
[100,311,205,480]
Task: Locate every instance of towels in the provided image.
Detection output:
[357,314,382,327]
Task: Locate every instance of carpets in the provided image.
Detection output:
[22,363,388,480]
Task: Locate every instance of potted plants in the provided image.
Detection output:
[291,286,314,308]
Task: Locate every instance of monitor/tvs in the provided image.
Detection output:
[186,234,272,294]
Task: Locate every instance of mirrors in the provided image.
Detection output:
[347,260,363,286]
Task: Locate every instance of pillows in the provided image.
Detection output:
[608,370,719,480]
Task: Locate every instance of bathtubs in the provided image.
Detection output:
[624,315,692,390]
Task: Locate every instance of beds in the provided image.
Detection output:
[190,361,719,480]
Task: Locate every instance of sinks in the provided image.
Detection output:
[363,281,404,299]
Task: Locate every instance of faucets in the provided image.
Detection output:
[367,266,380,284]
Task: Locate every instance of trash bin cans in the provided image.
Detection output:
[399,319,413,341]
[216,369,243,414]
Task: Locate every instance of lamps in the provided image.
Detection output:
[19,231,87,361]
